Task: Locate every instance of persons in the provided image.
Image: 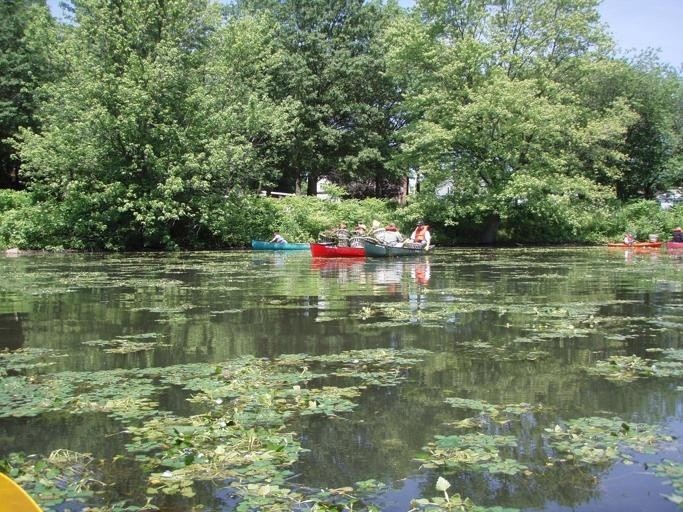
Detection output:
[669,228,683,242]
[356,225,366,237]
[277,238,288,244]
[383,223,404,246]
[269,232,282,242]
[624,233,637,244]
[406,220,431,252]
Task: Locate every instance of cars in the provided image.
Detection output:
[643,185,682,211]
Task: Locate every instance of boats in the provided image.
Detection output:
[251,250,308,262]
[309,240,365,258]
[664,240,681,254]
[250,232,309,250]
[310,256,368,272]
[365,257,426,275]
[604,233,663,250]
[348,236,436,257]
